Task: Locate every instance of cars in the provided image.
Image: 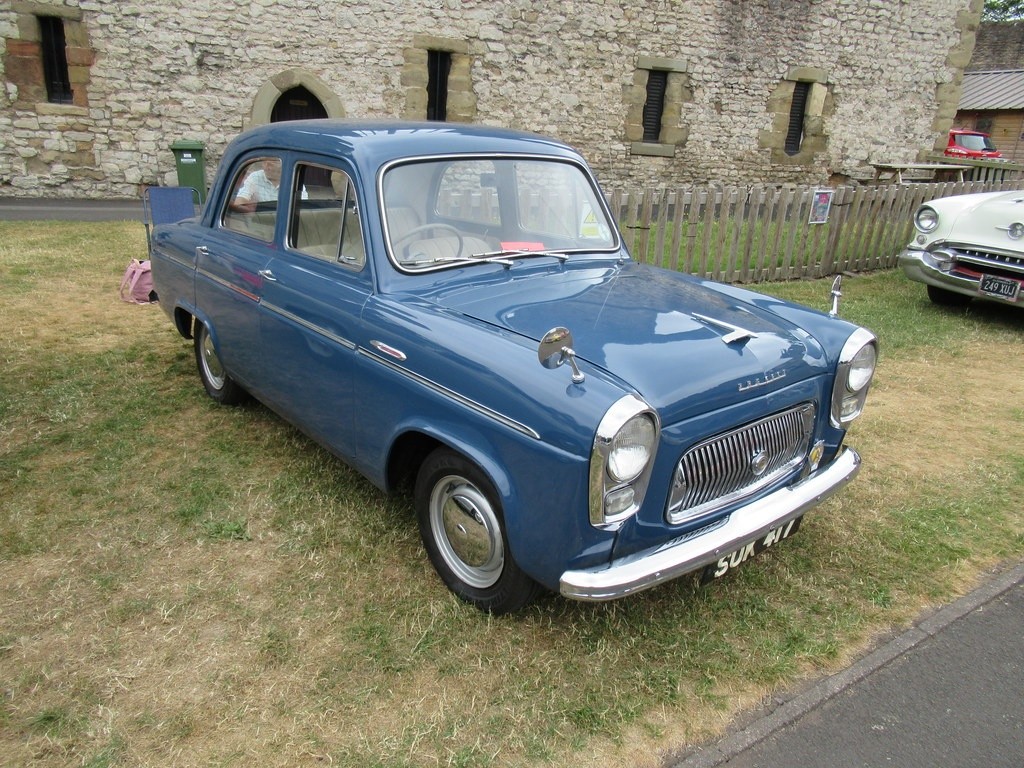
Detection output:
[933,127,1003,182]
[145,115,883,618]
[898,189,1024,308]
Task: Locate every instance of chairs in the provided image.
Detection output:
[405,237,492,265]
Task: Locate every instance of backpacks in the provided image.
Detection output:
[119,259,158,305]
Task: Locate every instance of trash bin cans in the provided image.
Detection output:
[168,138,207,205]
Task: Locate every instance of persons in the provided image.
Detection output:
[233,161,308,205]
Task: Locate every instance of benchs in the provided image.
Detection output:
[229,203,422,258]
